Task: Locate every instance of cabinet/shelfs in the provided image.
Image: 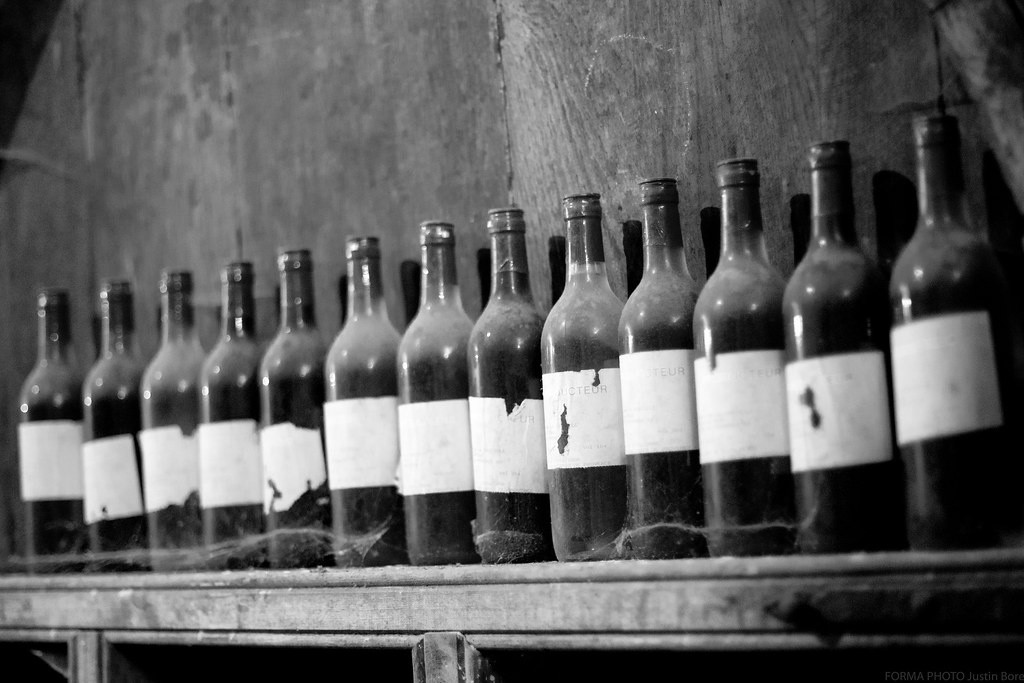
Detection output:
[0,552,1024,683]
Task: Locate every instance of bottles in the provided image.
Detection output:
[323,236,409,565]
[82,281,149,573]
[19,287,88,576]
[198,262,269,570]
[397,220,483,565]
[620,178,708,559]
[889,113,1023,550]
[139,270,207,572]
[694,159,798,558]
[261,250,332,567]
[467,206,557,563]
[782,139,911,555]
[541,193,630,561]
